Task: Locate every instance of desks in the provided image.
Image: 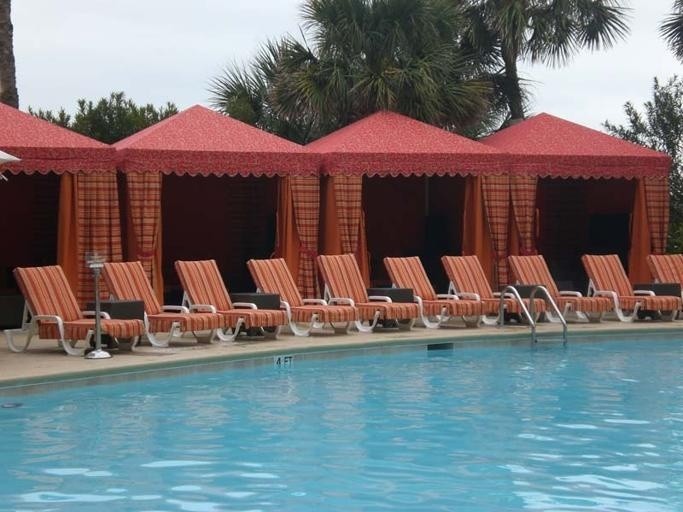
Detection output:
[366,287,414,326]
[85,299,144,353]
[229,293,280,335]
[498,286,546,325]
[635,283,681,320]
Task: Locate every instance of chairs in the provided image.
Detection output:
[98,261,226,348]
[440,255,545,325]
[246,258,359,335]
[647,253,682,285]
[173,259,288,344]
[582,255,682,325]
[507,255,612,325]
[317,255,421,331]
[2,264,146,354]
[382,255,486,329]
